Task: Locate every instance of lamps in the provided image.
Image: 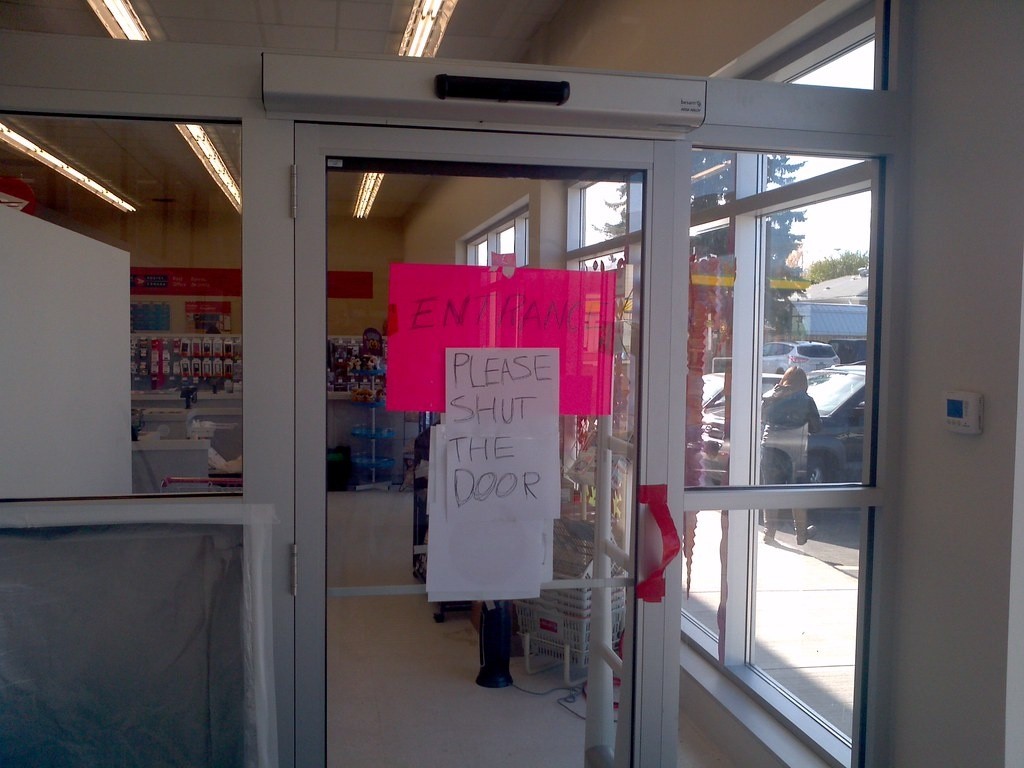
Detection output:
[0,0,459,214]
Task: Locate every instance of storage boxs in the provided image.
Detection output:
[131,336,244,380]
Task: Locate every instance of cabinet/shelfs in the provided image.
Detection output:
[349,400,397,491]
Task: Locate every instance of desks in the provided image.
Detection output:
[130,391,418,461]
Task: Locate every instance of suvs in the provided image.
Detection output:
[702,360,866,520]
[762,338,842,378]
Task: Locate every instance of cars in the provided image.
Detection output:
[701,371,787,409]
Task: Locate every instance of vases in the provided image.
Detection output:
[130,408,147,441]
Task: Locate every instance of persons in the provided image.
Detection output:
[758,365,824,545]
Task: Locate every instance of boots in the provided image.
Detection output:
[763,521,777,544]
[794,520,817,545]
